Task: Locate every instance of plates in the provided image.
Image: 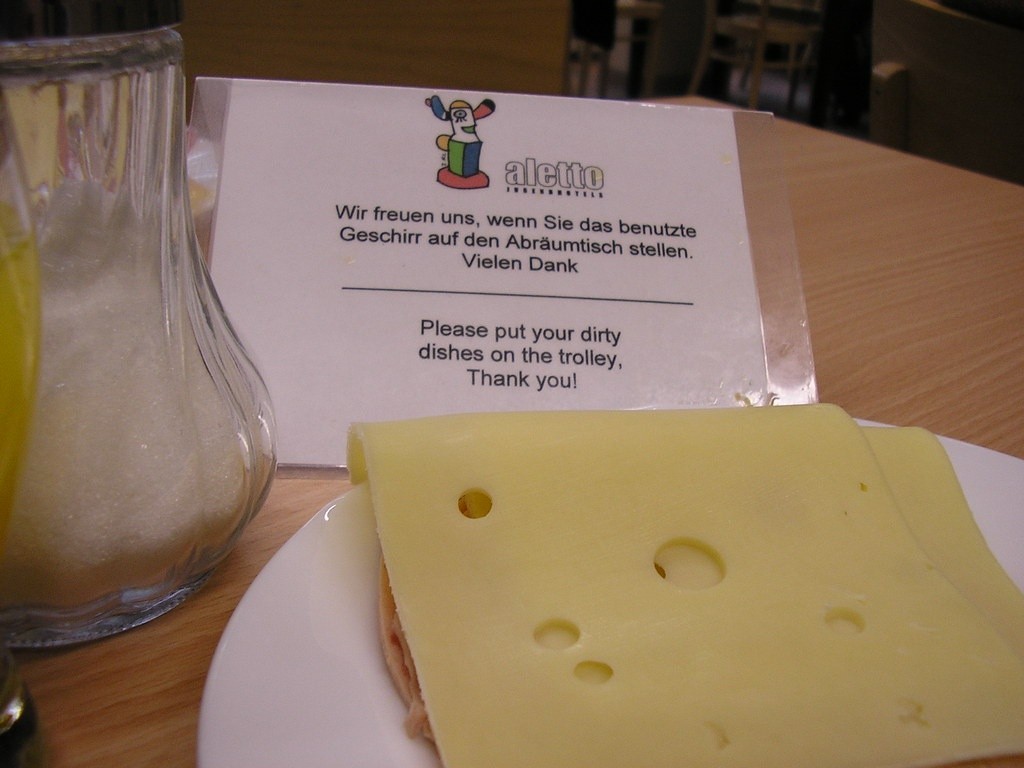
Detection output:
[193,411,1023,768]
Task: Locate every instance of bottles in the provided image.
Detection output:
[0,25,281,651]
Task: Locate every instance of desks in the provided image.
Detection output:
[0,89,1024,768]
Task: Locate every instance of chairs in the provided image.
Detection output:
[566,0,822,110]
